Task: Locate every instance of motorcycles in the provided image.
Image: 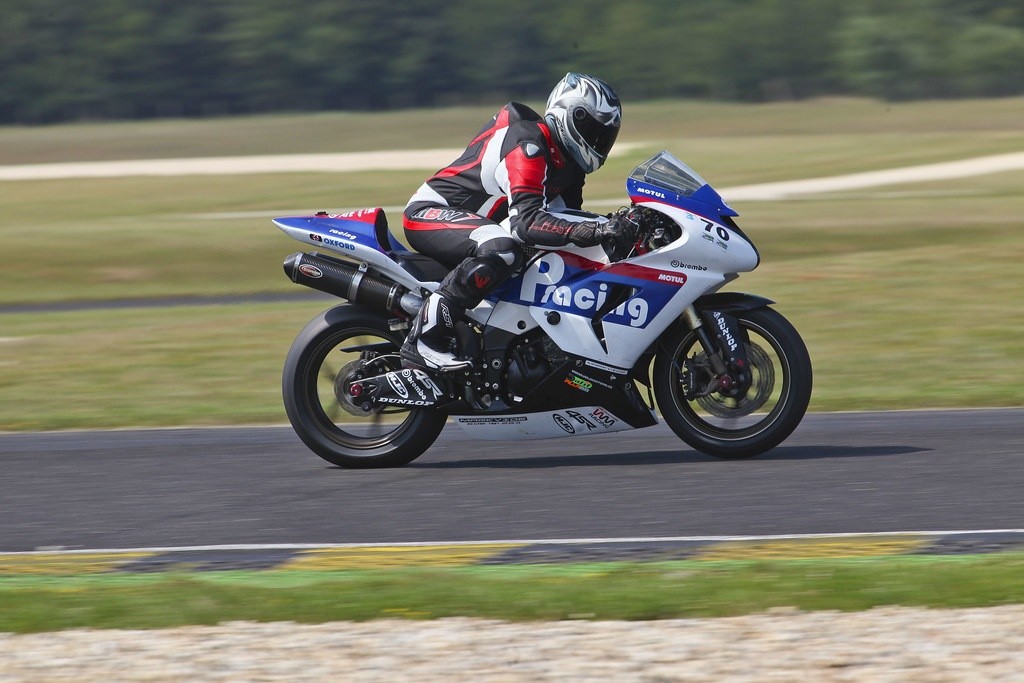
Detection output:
[268,148,815,473]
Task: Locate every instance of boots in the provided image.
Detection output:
[399,293,473,372]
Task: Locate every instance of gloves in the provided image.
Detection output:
[569,216,637,255]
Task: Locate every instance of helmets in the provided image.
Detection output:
[544,72,622,174]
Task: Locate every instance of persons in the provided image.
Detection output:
[399,70,636,376]
